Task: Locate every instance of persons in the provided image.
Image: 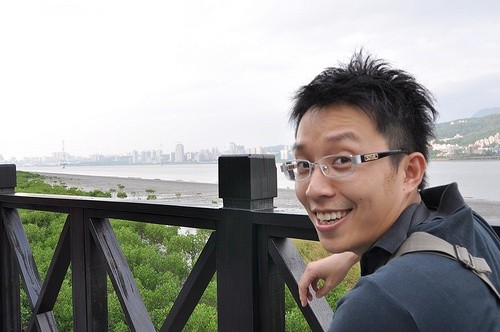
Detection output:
[289,51,500,332]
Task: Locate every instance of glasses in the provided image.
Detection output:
[279,149,411,182]
